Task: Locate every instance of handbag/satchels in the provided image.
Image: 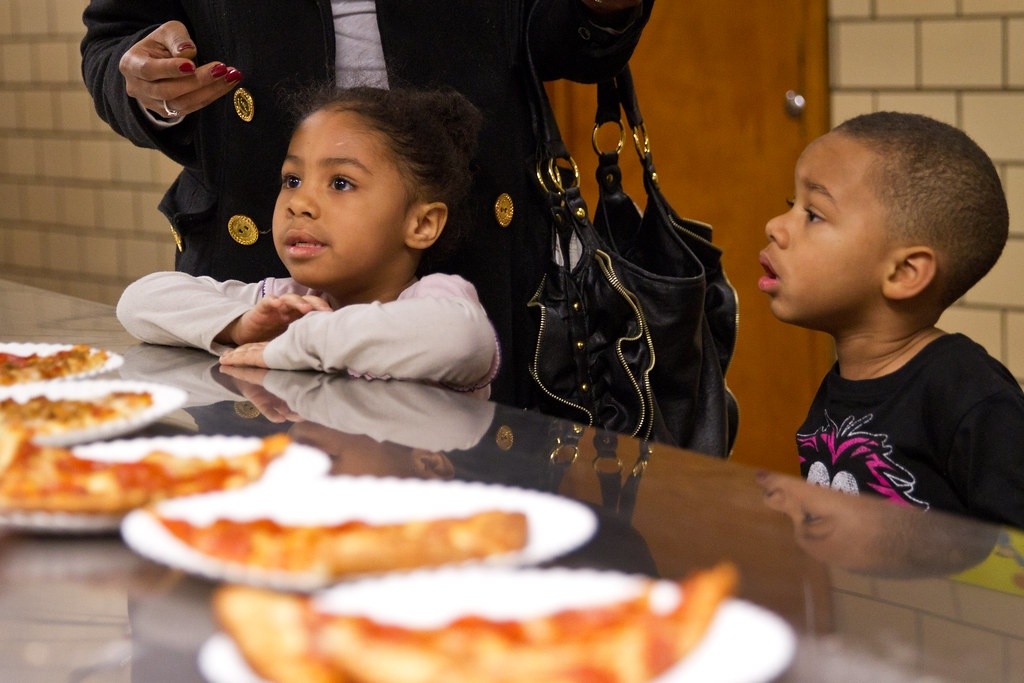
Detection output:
[503,1,739,459]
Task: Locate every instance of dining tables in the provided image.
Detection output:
[0,279,1024,683]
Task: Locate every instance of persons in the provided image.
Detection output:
[754,470,1002,579]
[79,0,654,413]
[756,110,1024,530]
[115,86,500,406]
[116,342,497,481]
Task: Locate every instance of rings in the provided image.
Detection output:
[163,99,180,117]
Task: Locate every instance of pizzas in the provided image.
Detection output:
[222,562,738,681]
[0,393,152,429]
[1,434,289,512]
[163,512,529,577]
[0,345,107,385]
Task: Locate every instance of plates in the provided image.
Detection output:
[0,380,186,444]
[0,435,333,530]
[196,570,793,683]
[0,343,124,385]
[122,475,596,590]
